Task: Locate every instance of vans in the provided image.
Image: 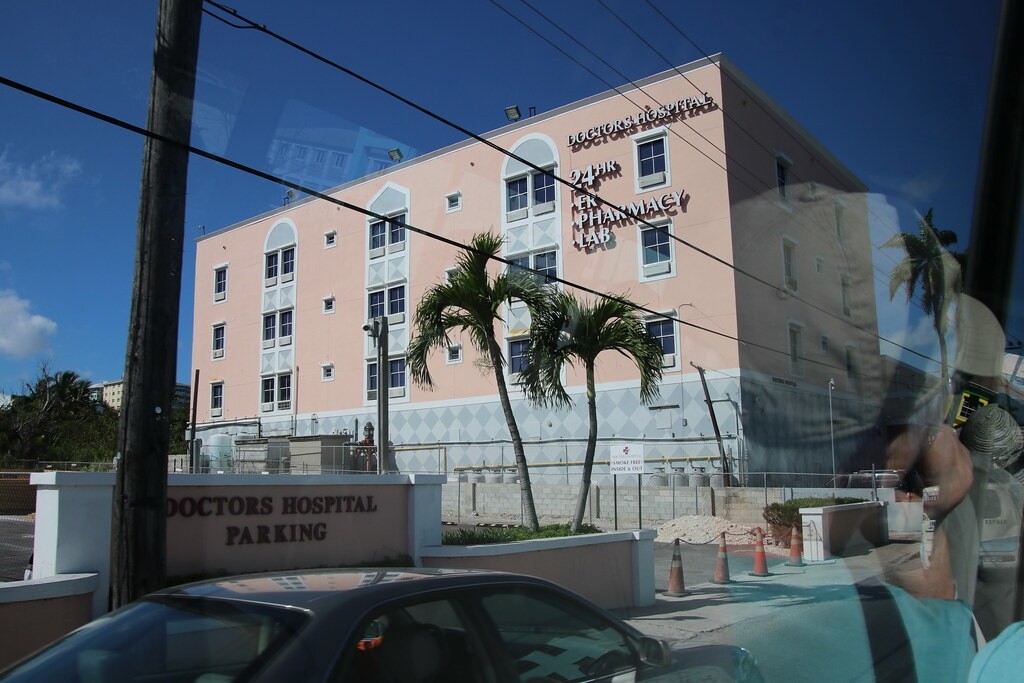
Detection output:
[918,483,1024,574]
[821,465,909,489]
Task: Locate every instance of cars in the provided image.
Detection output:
[1,564,764,683]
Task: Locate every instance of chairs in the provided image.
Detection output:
[378,623,447,683]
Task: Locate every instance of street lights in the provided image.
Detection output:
[827,376,839,499]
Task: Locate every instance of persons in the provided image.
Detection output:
[674,182,1024,683]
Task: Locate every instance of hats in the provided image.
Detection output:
[731,182,1006,447]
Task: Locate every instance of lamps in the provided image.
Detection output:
[287,189,298,202]
[388,148,403,163]
[199,224,205,235]
[504,105,521,122]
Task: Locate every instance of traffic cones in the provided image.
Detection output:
[785,524,806,568]
[661,536,692,599]
[705,532,736,585]
[745,527,775,577]
